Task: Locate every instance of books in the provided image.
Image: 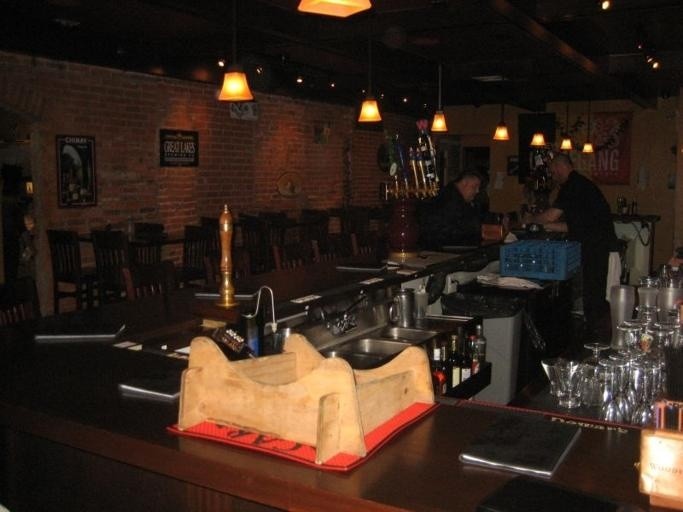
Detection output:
[193,284,259,299]
[440,241,477,250]
[33,318,125,342]
[335,262,388,273]
[457,406,583,479]
[116,359,181,401]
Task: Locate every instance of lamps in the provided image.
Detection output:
[528,90,595,154]
[492,77,510,142]
[429,62,450,132]
[355,28,383,124]
[293,1,374,19]
[216,0,255,104]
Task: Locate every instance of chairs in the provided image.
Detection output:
[0,274,43,327]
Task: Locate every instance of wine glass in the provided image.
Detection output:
[540,304,682,428]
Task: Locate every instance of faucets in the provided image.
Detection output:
[304,302,327,321]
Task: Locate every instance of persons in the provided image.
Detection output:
[414,169,485,253]
[517,152,619,342]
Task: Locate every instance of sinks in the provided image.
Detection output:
[338,335,413,359]
[370,325,437,346]
[316,349,383,370]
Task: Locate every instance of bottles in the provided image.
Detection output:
[429,324,486,396]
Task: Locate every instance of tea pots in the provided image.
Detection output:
[388,288,414,327]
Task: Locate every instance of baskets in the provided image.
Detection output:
[499,239,582,281]
